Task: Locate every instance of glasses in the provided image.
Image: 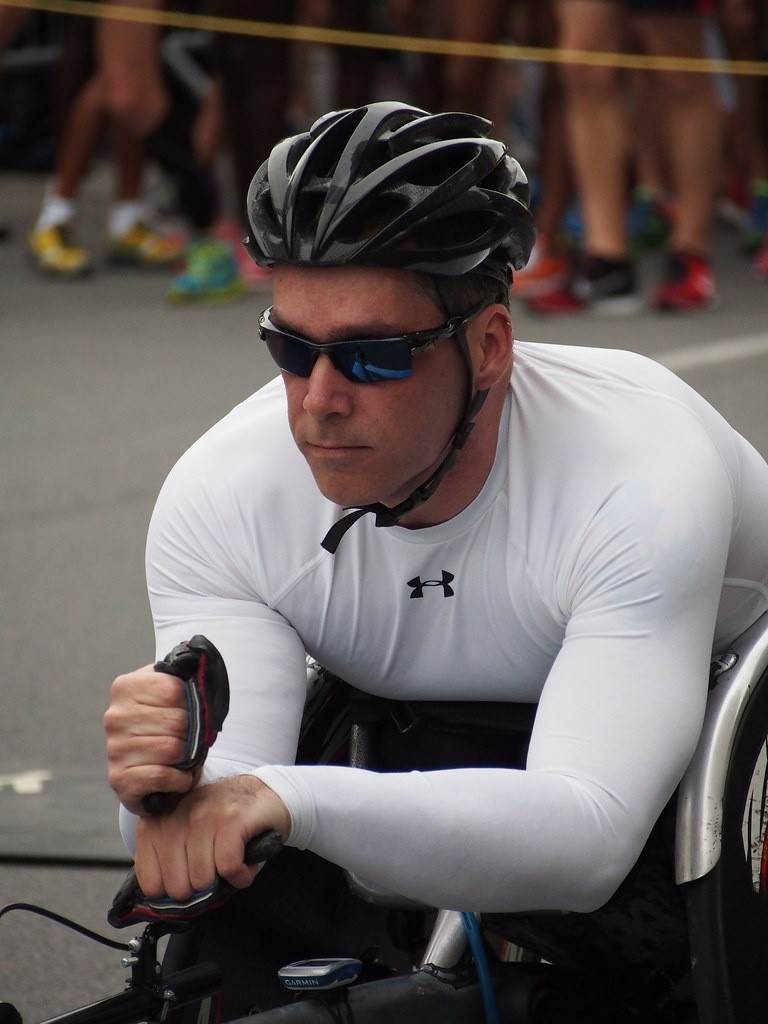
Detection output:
[257,299,500,382]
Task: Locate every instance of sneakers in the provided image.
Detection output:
[24,224,94,279]
[94,215,187,267]
[513,232,638,310]
[167,235,244,299]
[644,242,720,310]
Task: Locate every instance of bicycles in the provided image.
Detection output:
[1,604,768,1024]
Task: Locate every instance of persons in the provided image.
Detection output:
[104,96,768,1024]
[1,0,768,314]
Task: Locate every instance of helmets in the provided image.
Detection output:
[238,97,539,281]
